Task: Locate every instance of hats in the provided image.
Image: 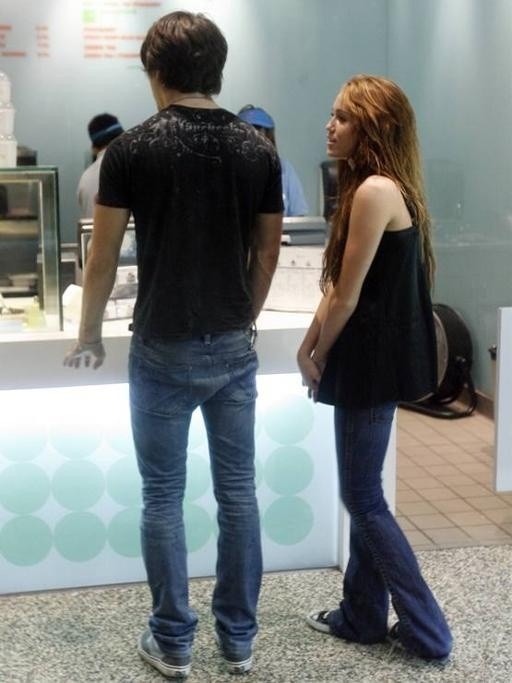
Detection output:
[239,106,274,129]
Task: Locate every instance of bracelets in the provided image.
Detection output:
[78,340,102,344]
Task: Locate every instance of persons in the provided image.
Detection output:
[298,76,459,666]
[63,11,284,680]
[77,112,133,224]
[239,104,308,215]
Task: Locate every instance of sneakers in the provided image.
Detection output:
[214,631,253,674]
[385,619,406,657]
[136,629,191,678]
[306,610,333,633]
